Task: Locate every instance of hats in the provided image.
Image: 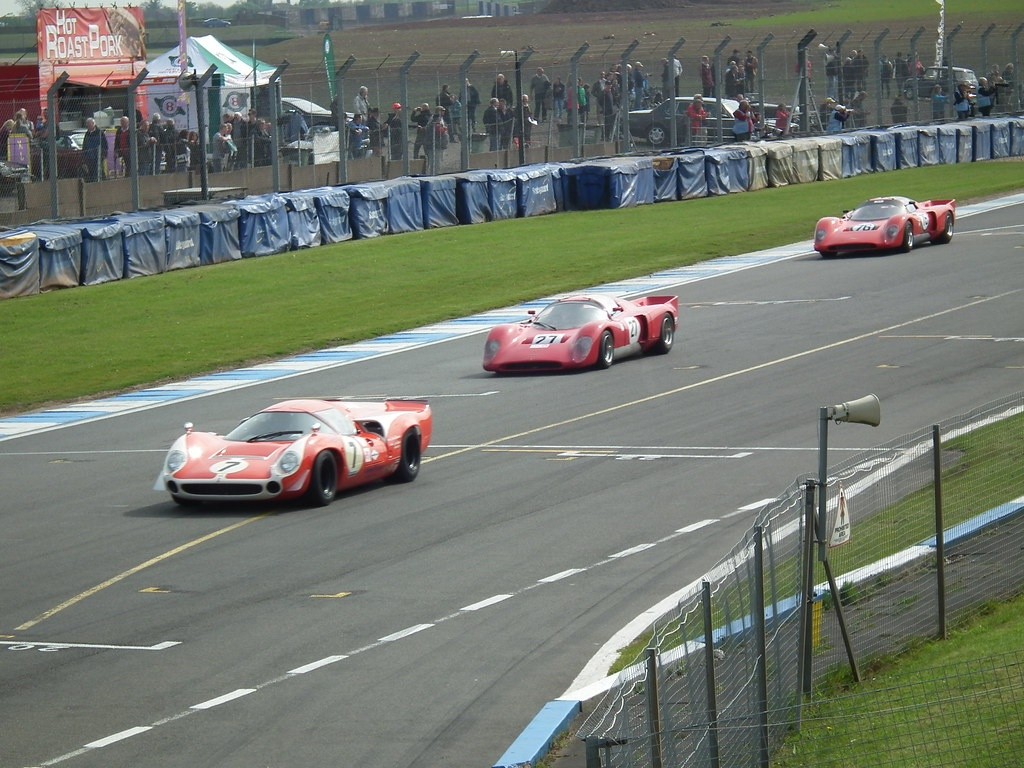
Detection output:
[835,105,846,110]
[825,97,835,103]
[392,103,402,112]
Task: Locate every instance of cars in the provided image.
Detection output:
[904,65,979,102]
[748,102,798,140]
[627,96,769,148]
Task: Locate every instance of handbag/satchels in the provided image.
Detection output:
[920,65,927,74]
[826,61,838,75]
[431,134,448,149]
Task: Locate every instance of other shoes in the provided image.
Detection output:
[534,117,547,123]
[450,140,458,144]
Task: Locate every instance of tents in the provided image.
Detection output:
[143,34,282,162]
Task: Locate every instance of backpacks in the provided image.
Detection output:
[880,59,890,80]
[901,61,909,75]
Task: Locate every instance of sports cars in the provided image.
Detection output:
[481,290,681,375]
[812,196,957,260]
[155,383,429,508]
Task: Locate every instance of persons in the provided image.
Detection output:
[819,46,925,134]
[931,84,950,120]
[795,57,812,87]
[775,104,791,132]
[0,48,760,179]
[423,106,449,176]
[953,63,1015,119]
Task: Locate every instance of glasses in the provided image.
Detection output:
[234,116,238,119]
